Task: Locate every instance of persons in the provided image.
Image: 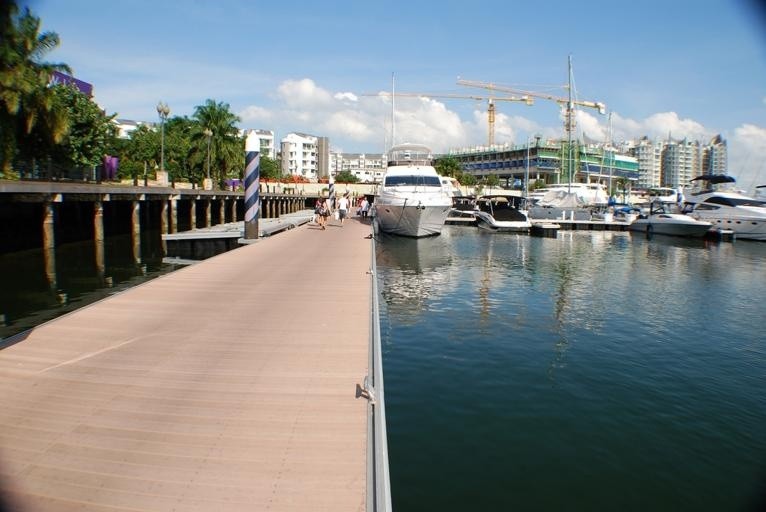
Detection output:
[336,194,350,227]
[360,196,369,220]
[319,198,328,230]
[315,196,322,226]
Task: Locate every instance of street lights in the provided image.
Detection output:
[204,128,213,179]
[156,101,170,171]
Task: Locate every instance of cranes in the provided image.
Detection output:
[455,55,607,184]
[363,83,536,153]
